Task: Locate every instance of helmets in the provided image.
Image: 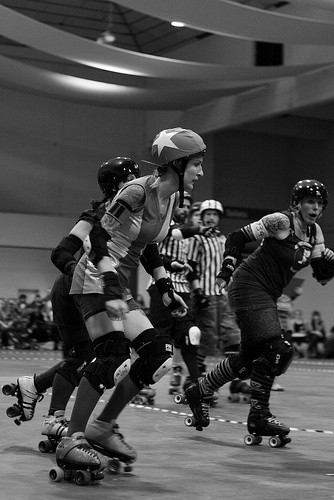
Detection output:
[151,127,206,168]
[292,180,328,207]
[200,199,224,217]
[188,202,202,218]
[177,191,193,205]
[97,156,140,200]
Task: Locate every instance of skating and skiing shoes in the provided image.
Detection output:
[2,372,46,426]
[168,382,184,395]
[228,380,252,404]
[271,377,284,392]
[244,407,292,447]
[38,410,71,453]
[130,387,156,405]
[48,432,104,486]
[83,419,137,472]
[174,380,210,431]
[200,390,218,407]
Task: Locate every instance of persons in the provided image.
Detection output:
[0,129,334,486]
